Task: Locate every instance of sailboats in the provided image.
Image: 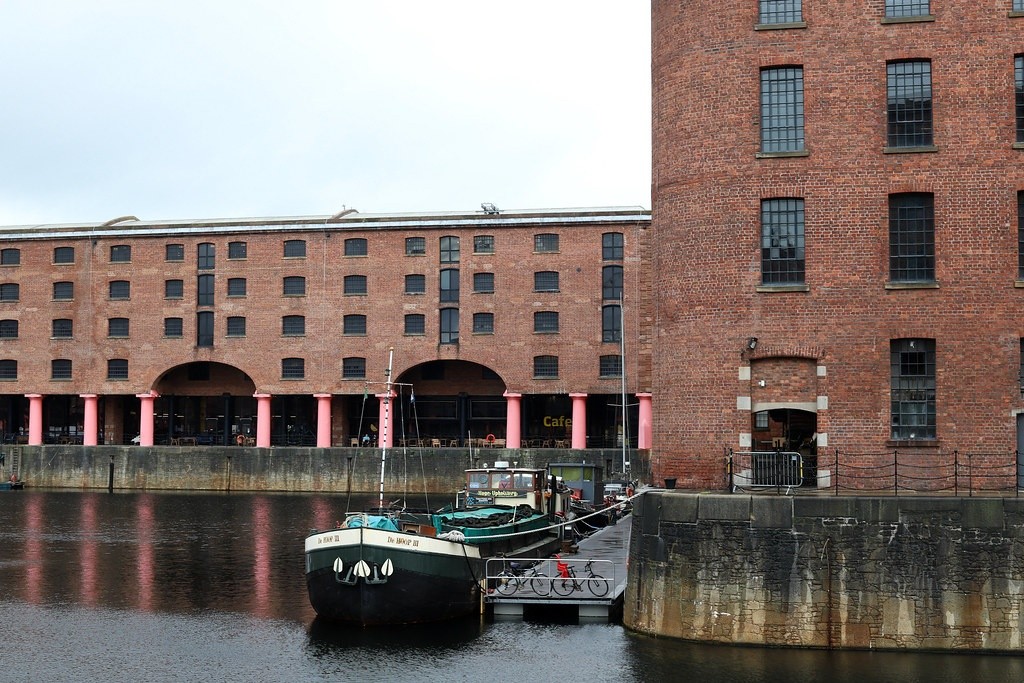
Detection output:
[602,291,647,516]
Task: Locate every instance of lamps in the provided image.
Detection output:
[749,337,759,350]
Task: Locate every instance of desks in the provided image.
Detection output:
[178,436,198,446]
[532,439,544,447]
[408,438,421,447]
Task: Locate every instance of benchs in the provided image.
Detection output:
[478,438,505,448]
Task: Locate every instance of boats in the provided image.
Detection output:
[304,347,481,617]
[545,460,611,529]
[429,431,570,574]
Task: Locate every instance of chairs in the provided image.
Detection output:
[521,438,571,449]
[170,436,178,446]
[350,438,358,447]
[398,438,484,447]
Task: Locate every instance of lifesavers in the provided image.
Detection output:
[628,488,634,497]
[237,435,245,443]
[486,434,495,443]
